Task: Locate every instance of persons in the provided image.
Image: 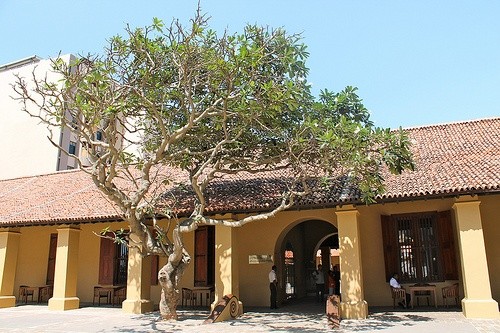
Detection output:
[328,270,335,295]
[390,272,411,309]
[269,265,279,309]
[312,264,324,304]
[332,265,340,295]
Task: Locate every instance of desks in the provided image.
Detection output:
[409,286,438,310]
[92,285,126,308]
[182,286,213,310]
[18,285,53,305]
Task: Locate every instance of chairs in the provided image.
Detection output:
[390,286,406,310]
[441,281,460,309]
